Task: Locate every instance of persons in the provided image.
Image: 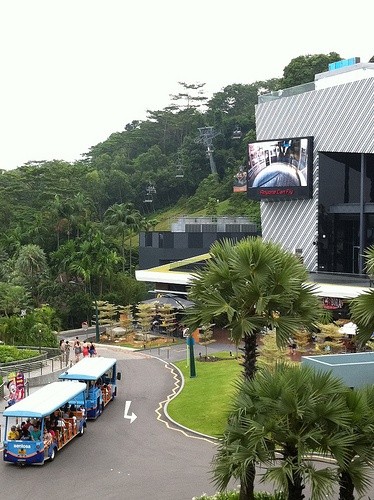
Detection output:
[8,401,86,449]
[73,337,80,350]
[64,341,71,361]
[74,340,81,363]
[82,341,89,357]
[95,373,111,396]
[59,339,65,351]
[88,341,97,358]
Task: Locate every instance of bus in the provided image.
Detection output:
[0,382,86,467]
[57,356,122,421]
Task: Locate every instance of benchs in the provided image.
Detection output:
[54,411,82,448]
